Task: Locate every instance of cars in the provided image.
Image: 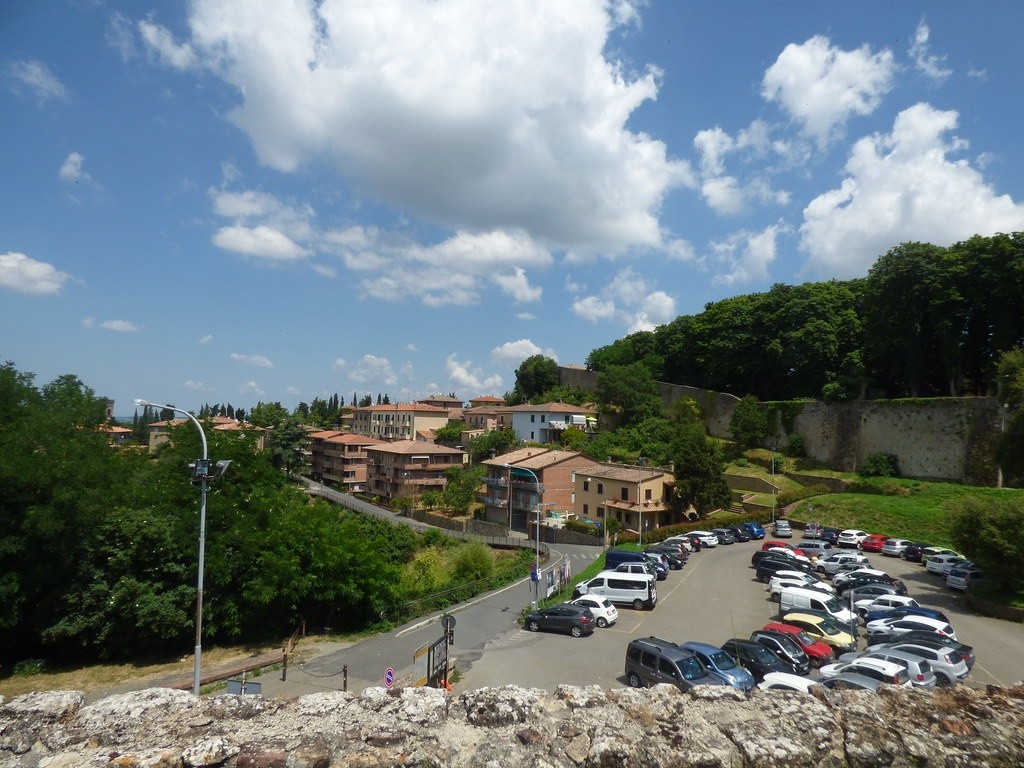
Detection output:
[564,594,620,628]
[603,561,658,581]
[643,522,736,572]
[722,522,983,696]
[774,519,792,537]
[679,641,755,692]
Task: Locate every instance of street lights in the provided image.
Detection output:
[586,477,607,552]
[503,463,540,611]
[404,472,415,516]
[130,397,210,695]
[340,454,352,495]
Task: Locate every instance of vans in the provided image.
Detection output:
[572,570,658,611]
[606,549,669,580]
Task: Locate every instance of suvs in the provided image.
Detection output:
[624,635,725,691]
[522,594,620,636]
